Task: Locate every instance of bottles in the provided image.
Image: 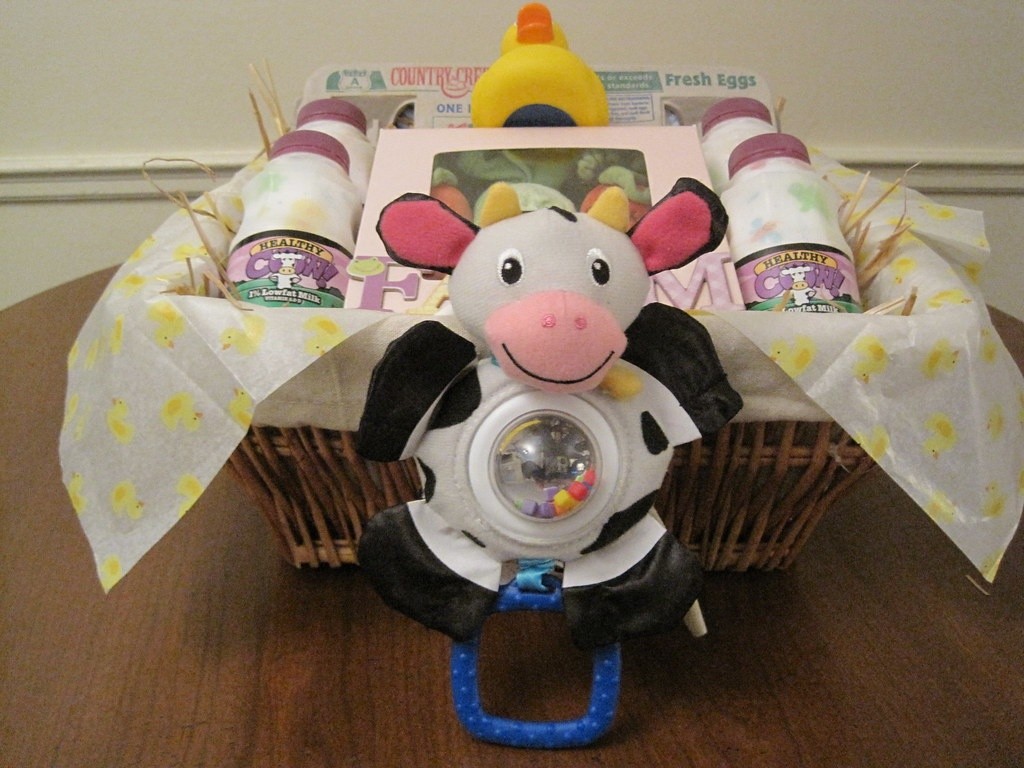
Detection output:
[222,130,355,309]
[294,99,374,242]
[701,97,778,199]
[719,133,864,313]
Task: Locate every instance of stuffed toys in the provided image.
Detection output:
[354,177,744,654]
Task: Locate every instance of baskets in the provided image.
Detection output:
[221,420,876,577]
